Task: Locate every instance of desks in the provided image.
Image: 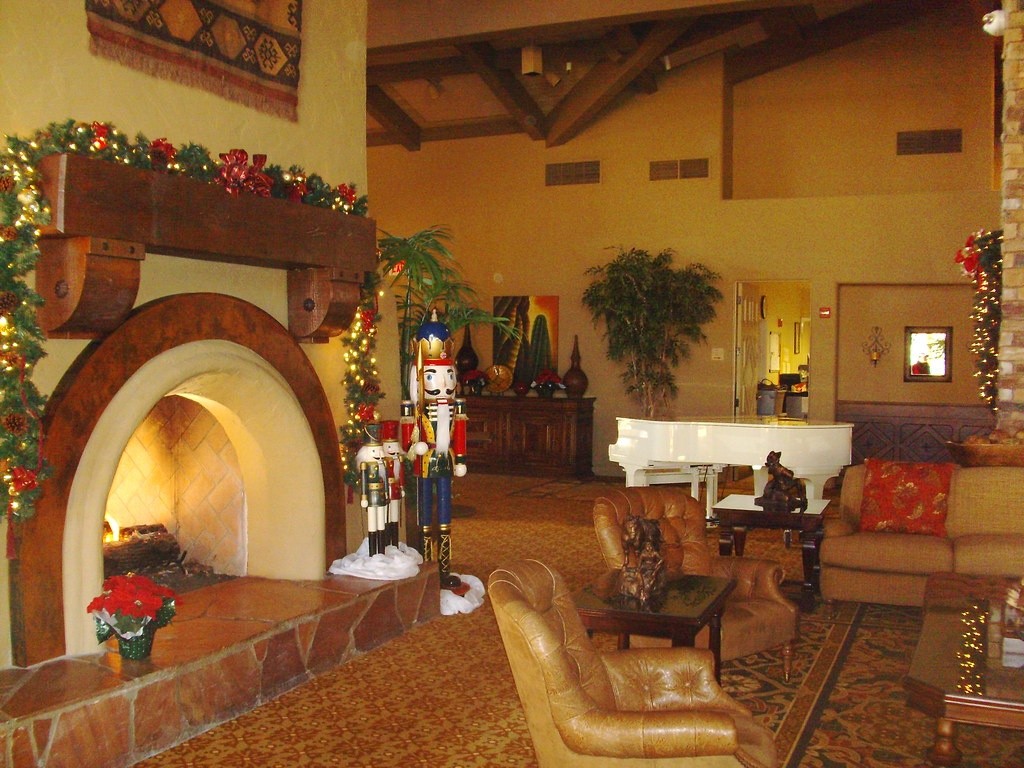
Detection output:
[711,494,831,610]
[569,568,737,689]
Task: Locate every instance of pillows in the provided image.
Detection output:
[859,459,954,538]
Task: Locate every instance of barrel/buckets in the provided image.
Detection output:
[757,390,776,415]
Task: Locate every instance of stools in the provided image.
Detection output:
[622,467,718,528]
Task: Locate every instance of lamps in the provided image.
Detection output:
[521,39,543,78]
[545,70,562,87]
[427,79,441,100]
[861,326,892,367]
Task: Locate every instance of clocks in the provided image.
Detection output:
[761,295,767,319]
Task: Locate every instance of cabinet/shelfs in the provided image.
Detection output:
[457,395,597,480]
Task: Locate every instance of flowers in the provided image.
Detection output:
[86,573,185,634]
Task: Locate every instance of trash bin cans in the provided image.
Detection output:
[758,383,776,415]
[760,385,788,414]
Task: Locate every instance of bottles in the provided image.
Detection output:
[563,334,588,396]
[539,369,557,397]
[455,325,482,397]
[514,381,529,398]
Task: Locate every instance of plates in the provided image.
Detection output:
[484,366,512,393]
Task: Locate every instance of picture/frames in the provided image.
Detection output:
[795,322,800,354]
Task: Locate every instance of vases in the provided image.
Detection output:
[116,614,156,659]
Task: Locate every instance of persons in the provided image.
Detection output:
[356,423,390,557]
[401,309,468,588]
[638,541,665,575]
[764,451,793,498]
[379,419,406,554]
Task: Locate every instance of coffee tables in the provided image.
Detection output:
[903,573,1024,768]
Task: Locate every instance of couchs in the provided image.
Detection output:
[799,457,1024,608]
[485,558,778,768]
[594,485,801,686]
